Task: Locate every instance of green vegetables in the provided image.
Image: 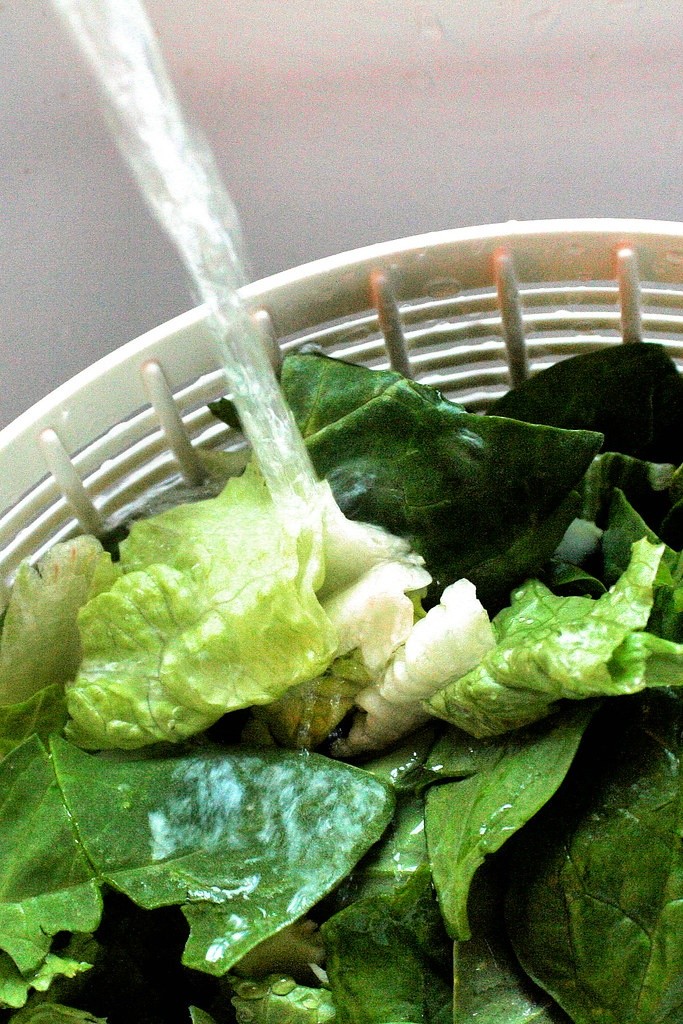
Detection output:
[0,340,683,1024]
